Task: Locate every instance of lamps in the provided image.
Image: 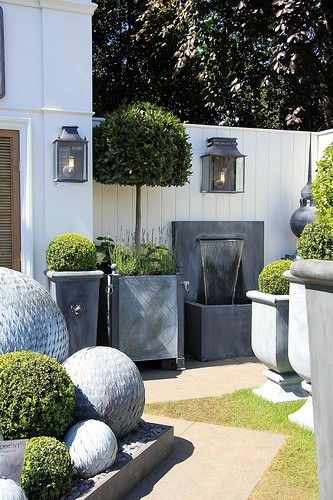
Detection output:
[53,126,89,183]
[200,137,247,194]
[93,102,192,371]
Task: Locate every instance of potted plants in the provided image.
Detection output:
[44,233,104,358]
[283,143,333,431]
[246,259,309,403]
